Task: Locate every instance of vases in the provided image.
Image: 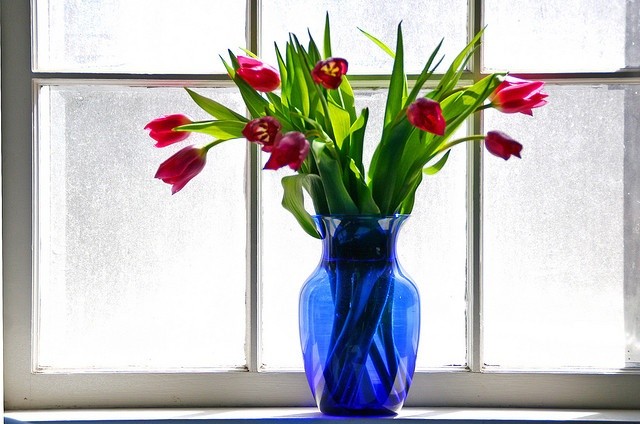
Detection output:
[298,214,421,417]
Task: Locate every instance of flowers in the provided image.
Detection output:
[143,10,549,224]
